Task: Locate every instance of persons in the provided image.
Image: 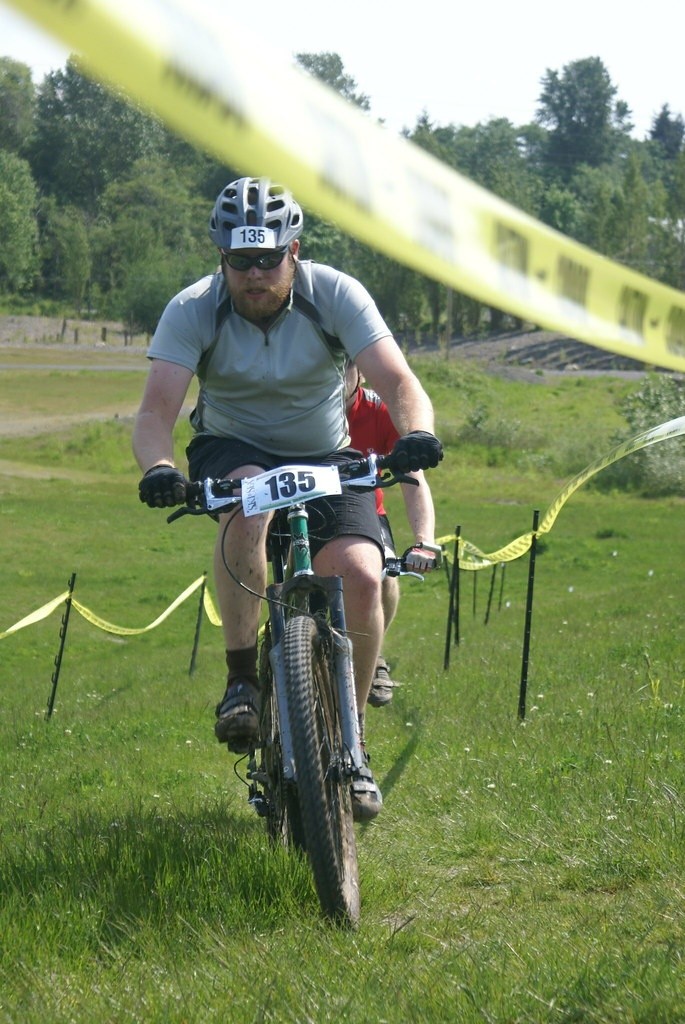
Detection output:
[130,178,445,821]
[344,357,439,708]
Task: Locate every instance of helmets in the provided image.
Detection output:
[208,177,304,252]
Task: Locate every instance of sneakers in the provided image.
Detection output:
[349,754,382,821]
[215,677,262,744]
[366,653,393,707]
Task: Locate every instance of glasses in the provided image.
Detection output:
[221,245,291,272]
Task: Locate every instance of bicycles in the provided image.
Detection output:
[138,446,447,934]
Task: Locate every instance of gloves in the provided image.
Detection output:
[389,430,443,475]
[138,464,189,508]
